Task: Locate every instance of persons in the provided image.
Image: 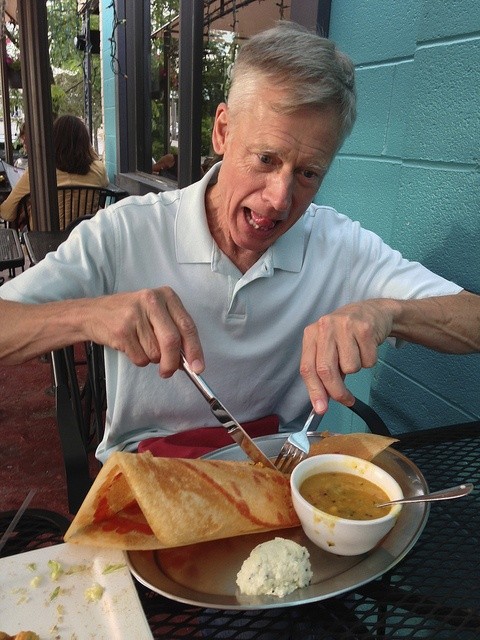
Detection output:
[153,154,178,179]
[14,123,32,172]
[0,23,480,467]
[1,114,109,232]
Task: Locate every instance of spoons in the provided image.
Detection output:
[365,480,473,510]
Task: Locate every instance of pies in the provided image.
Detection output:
[63,434,399,553]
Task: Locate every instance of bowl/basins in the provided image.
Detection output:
[289,451,405,557]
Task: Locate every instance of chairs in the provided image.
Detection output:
[15,185,131,230]
[64,214,96,229]
[49,322,109,513]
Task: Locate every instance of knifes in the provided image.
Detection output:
[176,349,278,473]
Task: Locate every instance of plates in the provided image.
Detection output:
[123,431,432,609]
[0,542,155,640]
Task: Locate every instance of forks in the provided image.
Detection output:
[272,408,318,475]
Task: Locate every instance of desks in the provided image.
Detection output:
[0,228,24,270]
